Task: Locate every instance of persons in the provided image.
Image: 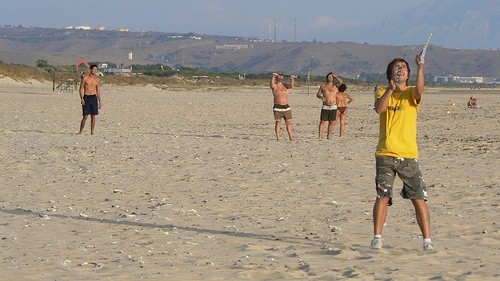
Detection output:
[336,83,355,137]
[77,64,104,135]
[467,96,480,108]
[81,72,87,79]
[370,54,435,251]
[270,72,297,142]
[316,72,343,140]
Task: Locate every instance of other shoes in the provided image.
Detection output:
[423,242,434,251]
[370,238,382,249]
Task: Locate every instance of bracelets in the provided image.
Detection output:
[80,97,84,99]
